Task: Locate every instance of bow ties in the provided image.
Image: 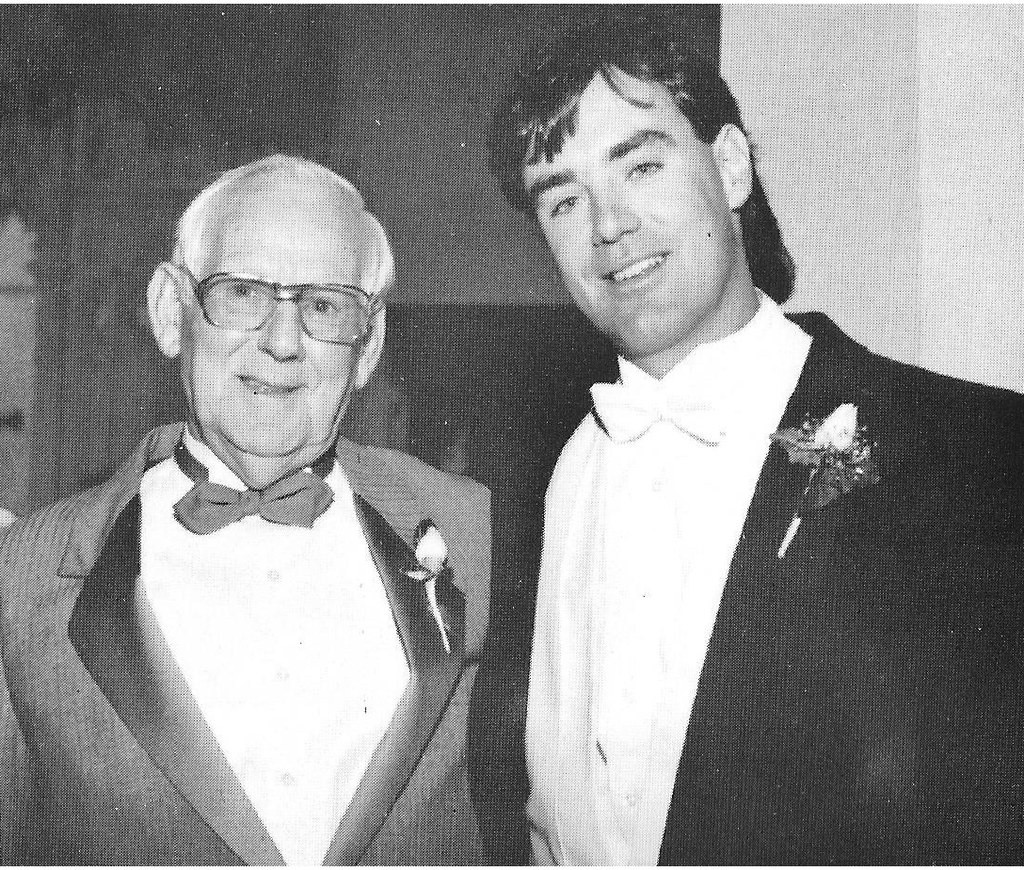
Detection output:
[172,437,337,535]
[588,380,732,446]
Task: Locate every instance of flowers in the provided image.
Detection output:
[401,520,462,656]
[771,403,886,561]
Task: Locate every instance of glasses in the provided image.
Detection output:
[180,264,373,347]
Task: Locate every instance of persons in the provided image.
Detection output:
[469,18,1024,866]
[0,154,543,866]
